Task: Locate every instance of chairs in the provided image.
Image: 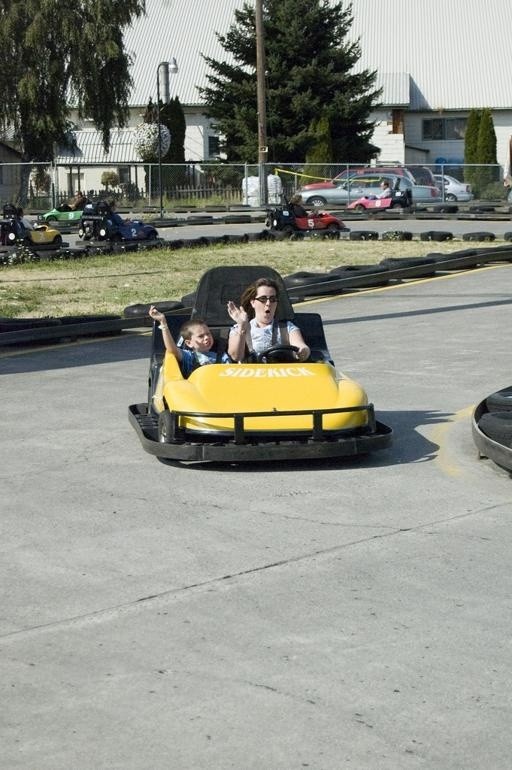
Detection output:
[191,265,294,353]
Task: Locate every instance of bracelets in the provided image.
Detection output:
[234,328,247,335]
[158,325,169,330]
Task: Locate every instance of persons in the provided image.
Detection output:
[61,191,83,211]
[107,198,131,226]
[368,181,393,200]
[226,278,312,365]
[291,195,316,218]
[149,304,233,379]
[17,208,38,231]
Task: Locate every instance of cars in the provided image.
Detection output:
[293,167,474,206]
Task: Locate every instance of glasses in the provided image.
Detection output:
[253,296,277,302]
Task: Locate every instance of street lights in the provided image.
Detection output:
[157,58,178,219]
